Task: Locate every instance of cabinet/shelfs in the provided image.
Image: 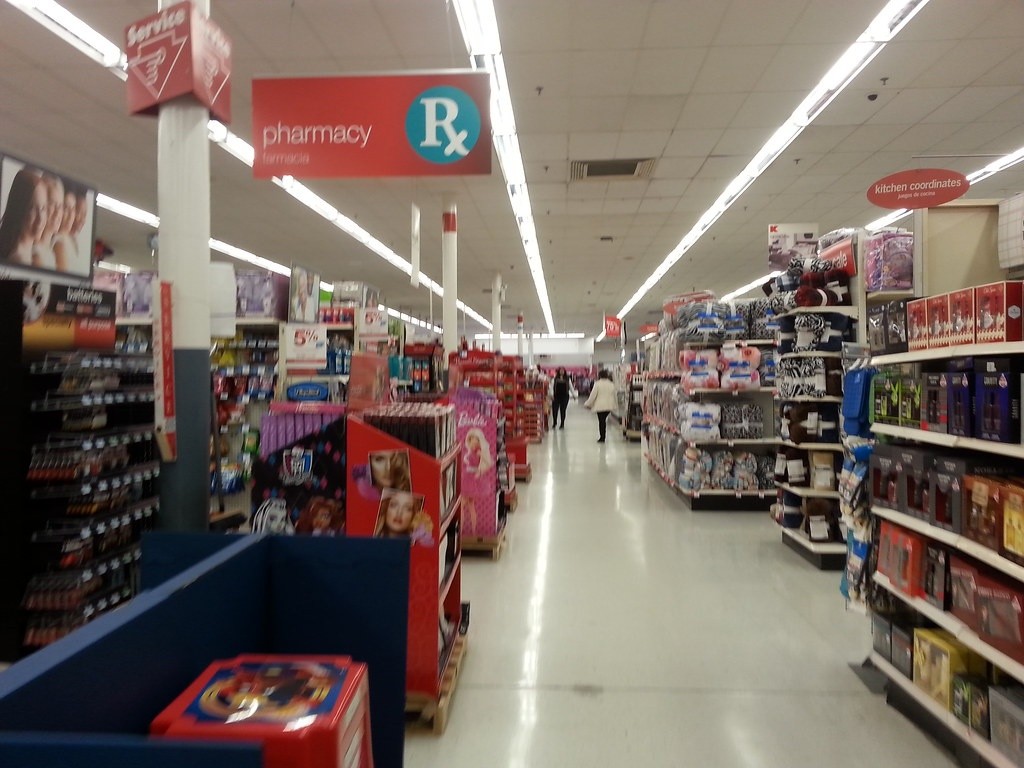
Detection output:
[608,229,914,767]
[858,345,1024,768]
[0,267,547,768]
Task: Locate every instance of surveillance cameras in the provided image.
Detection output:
[868,94,877,101]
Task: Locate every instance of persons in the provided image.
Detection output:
[368,448,425,545]
[1,167,90,273]
[584,370,616,442]
[548,367,577,431]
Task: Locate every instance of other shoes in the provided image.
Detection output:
[559,425,564,428]
[597,438,605,442]
[552,423,557,427]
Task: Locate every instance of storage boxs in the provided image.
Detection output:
[861,279,1023,768]
